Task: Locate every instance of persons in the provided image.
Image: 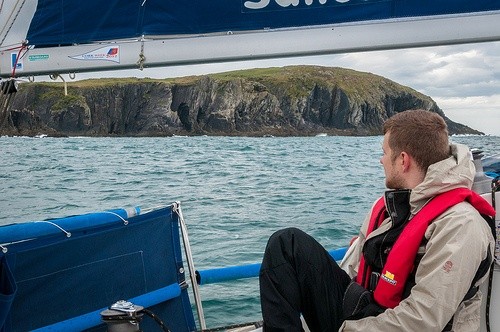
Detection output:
[259,109,496,332]
